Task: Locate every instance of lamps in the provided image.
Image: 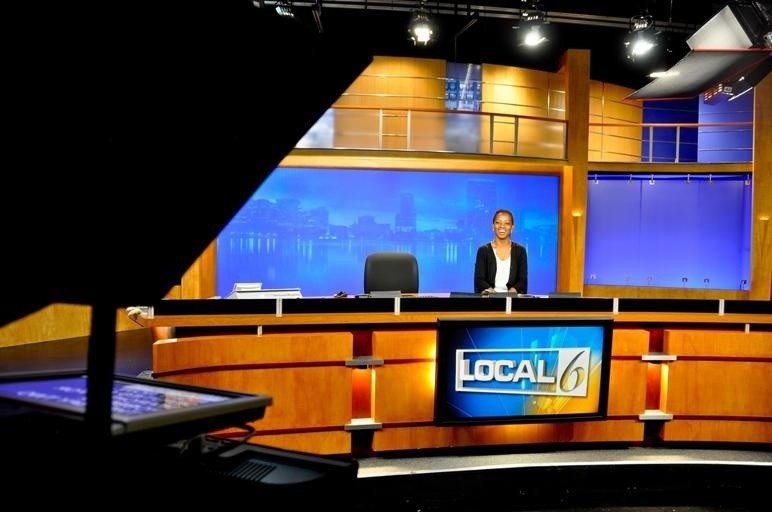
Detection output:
[403,3,661,59]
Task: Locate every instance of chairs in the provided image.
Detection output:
[364,252,419,294]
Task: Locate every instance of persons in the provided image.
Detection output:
[474,208,527,294]
